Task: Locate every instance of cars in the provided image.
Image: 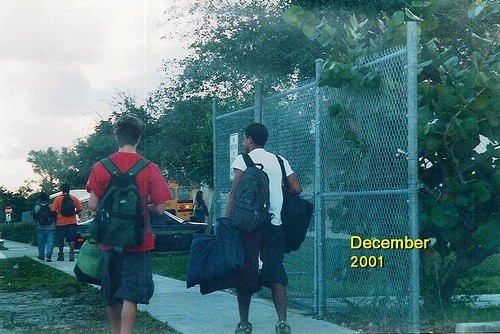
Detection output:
[74,210,214,253]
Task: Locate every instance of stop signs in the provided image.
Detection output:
[5,206,13,214]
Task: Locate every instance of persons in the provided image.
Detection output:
[50,184,83,261]
[86,116,172,334]
[33,193,56,262]
[192,191,208,223]
[225,123,303,334]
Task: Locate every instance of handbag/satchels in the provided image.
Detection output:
[75,237,106,287]
[197,204,207,215]
[276,155,314,253]
[187,218,245,288]
[200,273,245,295]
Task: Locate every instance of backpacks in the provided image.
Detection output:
[230,152,274,233]
[36,203,54,225]
[57,194,75,216]
[89,156,151,247]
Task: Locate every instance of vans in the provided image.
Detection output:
[164,181,193,222]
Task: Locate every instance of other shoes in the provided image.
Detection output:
[235,322,252,334]
[69,252,74,262]
[46,256,52,262]
[57,252,64,261]
[38,256,44,260]
[274,321,291,334]
